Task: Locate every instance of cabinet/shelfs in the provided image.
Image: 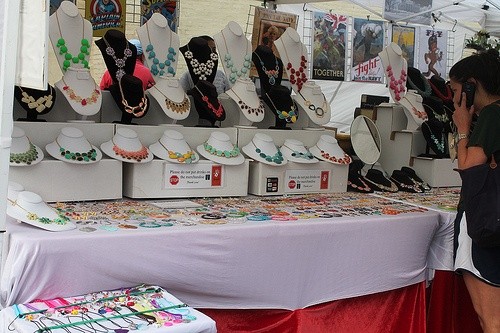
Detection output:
[9,87,348,202]
[376,107,462,187]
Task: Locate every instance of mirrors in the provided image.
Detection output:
[350,115,381,164]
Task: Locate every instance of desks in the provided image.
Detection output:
[369,188,483,333]
[0,285,216,333]
[0,190,440,333]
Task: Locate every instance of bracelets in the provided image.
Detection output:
[458,133,469,141]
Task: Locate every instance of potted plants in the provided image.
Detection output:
[463,28,490,54]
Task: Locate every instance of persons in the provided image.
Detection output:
[448,54,500,333]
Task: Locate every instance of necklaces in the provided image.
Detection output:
[14,284,196,333]
[379,46,458,158]
[349,172,430,194]
[8,10,351,228]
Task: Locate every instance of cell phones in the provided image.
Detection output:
[459,81,475,108]
